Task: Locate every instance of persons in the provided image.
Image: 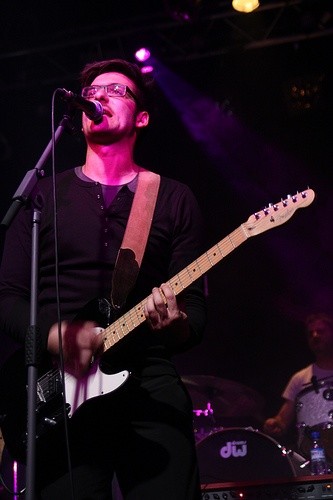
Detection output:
[1,58,202,500]
[261,311,333,472]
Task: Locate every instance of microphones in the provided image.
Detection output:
[61,88,103,121]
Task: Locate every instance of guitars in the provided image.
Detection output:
[1,185,317,467]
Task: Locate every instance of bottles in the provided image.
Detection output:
[309,432,327,476]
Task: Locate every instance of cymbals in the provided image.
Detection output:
[179,373,267,419]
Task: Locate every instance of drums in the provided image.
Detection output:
[194,426,298,482]
